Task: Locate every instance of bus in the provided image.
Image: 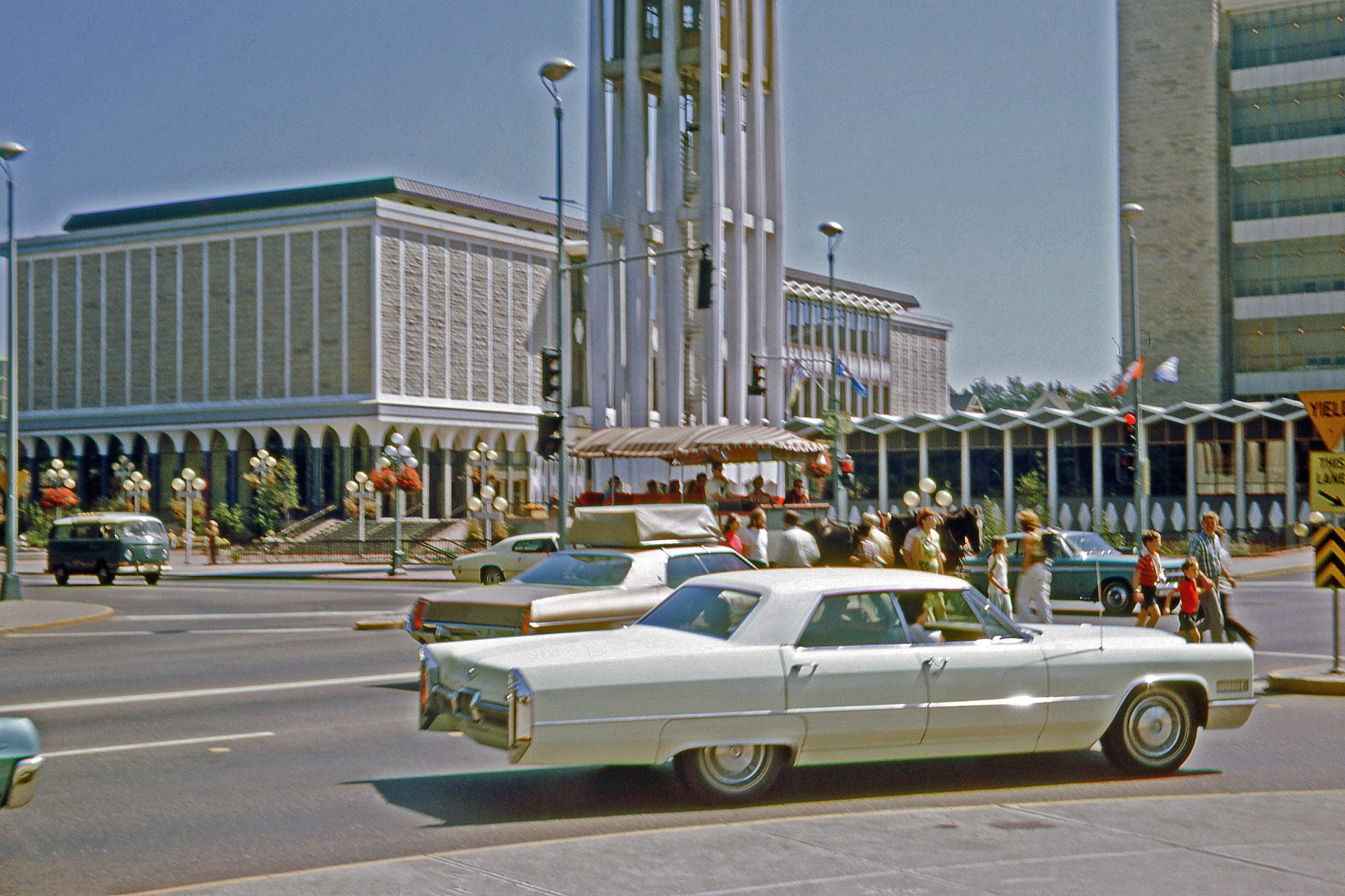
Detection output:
[47,511,171,586]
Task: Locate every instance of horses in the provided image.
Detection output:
[883,501,983,606]
[802,517,870,568]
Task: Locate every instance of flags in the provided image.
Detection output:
[1109,358,1143,398]
[836,357,867,398]
[787,360,812,409]
[1154,355,1179,384]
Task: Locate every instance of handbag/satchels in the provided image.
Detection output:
[1041,530,1064,559]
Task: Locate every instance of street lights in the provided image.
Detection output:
[0,140,27,599]
[1293,511,1345,673]
[1119,200,1147,553]
[171,468,207,566]
[815,221,844,527]
[534,55,578,545]
[345,431,509,577]
[249,449,277,483]
[122,469,152,512]
[44,457,77,519]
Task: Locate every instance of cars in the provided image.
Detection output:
[963,529,1189,617]
[1,716,44,812]
[416,567,1258,811]
[405,543,765,647]
[451,530,590,586]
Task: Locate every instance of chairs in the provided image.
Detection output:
[703,598,731,630]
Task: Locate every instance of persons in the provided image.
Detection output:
[167,520,220,564]
[604,461,1052,646]
[1132,529,1165,627]
[1162,511,1237,644]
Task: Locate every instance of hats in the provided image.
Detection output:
[782,511,804,527]
[916,507,935,521]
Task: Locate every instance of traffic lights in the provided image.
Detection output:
[536,410,565,461]
[751,364,767,396]
[1120,453,1136,477]
[840,459,856,487]
[1122,410,1137,446]
[540,345,562,405]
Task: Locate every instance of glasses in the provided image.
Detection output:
[795,485,803,489]
